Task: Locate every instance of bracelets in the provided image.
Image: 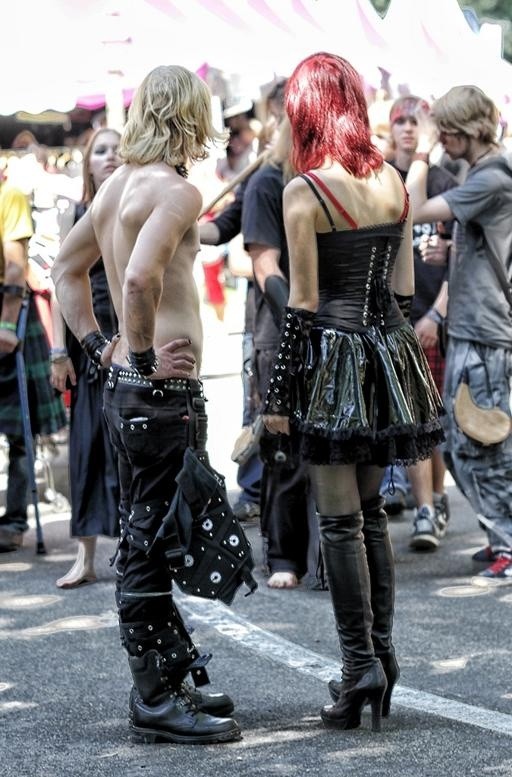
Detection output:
[431,307,443,320]
[426,309,442,324]
[1,321,16,330]
[49,348,68,354]
[49,356,68,363]
[79,329,110,366]
[410,151,428,164]
[127,345,160,378]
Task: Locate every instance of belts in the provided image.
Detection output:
[108,366,203,392]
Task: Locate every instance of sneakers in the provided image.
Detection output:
[383,488,511,577]
[233,499,261,522]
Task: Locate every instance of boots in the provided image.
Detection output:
[314,505,388,733]
[328,495,400,716]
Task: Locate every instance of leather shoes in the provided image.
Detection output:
[128,681,235,730]
[130,691,243,745]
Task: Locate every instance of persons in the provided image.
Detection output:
[0,51,512,593]
[48,62,239,742]
[259,52,446,739]
